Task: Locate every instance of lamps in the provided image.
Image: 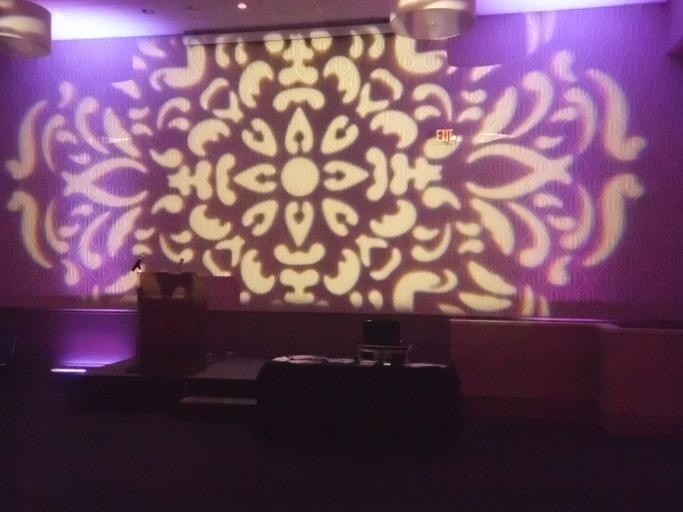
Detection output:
[181,17,397,47]
[389,0,476,41]
[0,0,51,58]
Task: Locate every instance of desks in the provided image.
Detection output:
[253,354,463,456]
